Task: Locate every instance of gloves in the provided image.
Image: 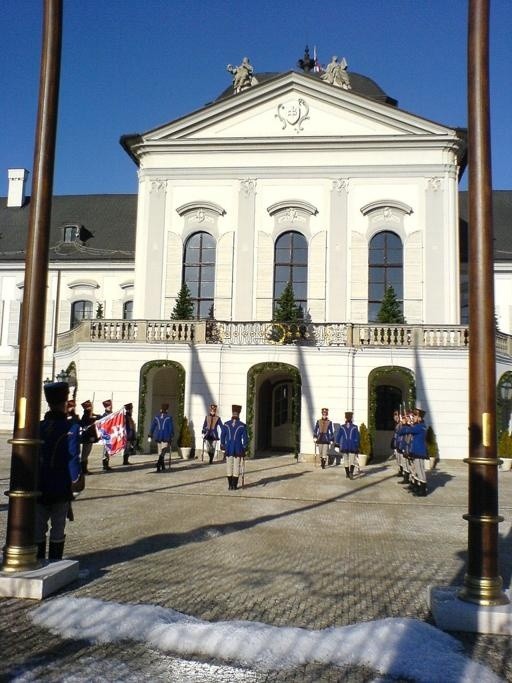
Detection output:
[313,438,317,443]
[334,447,340,453]
[73,491,80,498]
[330,441,334,445]
[148,437,152,443]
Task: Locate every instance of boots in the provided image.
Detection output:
[345,466,349,479]
[227,475,233,490]
[349,465,354,479]
[321,458,327,468]
[232,475,238,490]
[157,455,162,472]
[81,460,92,474]
[210,452,214,463]
[397,464,427,496]
[123,454,131,464]
[162,457,165,470]
[102,459,111,469]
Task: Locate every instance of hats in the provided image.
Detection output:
[394,407,424,418]
[124,402,133,409]
[44,383,70,404]
[232,404,242,412]
[345,412,353,417]
[322,408,328,413]
[68,400,77,407]
[161,403,169,409]
[211,404,217,409]
[103,400,113,407]
[81,399,92,408]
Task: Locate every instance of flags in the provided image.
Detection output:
[96,407,127,456]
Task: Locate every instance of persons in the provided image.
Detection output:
[390,400,429,496]
[335,410,360,480]
[319,55,352,88]
[123,402,137,464]
[81,399,105,473]
[148,402,174,472]
[37,382,83,559]
[66,399,83,425]
[101,398,113,470]
[312,407,334,468]
[227,55,254,89]
[220,404,248,490]
[202,404,223,463]
